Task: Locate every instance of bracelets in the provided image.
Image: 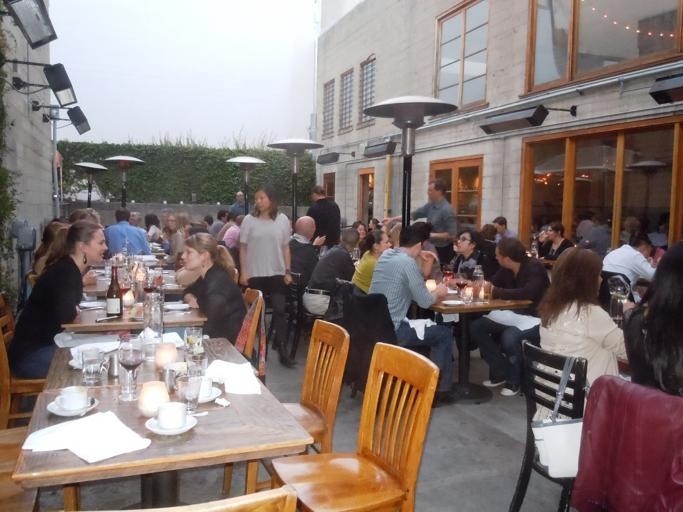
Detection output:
[285,268,292,274]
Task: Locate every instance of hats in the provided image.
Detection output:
[399,231,420,247]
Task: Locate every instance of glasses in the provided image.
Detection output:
[458,237,472,242]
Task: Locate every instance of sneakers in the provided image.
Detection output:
[483,377,506,386]
[500,382,520,396]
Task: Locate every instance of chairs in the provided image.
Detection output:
[224,321,351,496]
[510,341,588,512]
[268,272,312,365]
[342,287,432,398]
[272,342,440,512]
[234,289,264,357]
[0,290,57,429]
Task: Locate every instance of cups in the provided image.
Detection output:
[159,403,188,429]
[186,354,208,378]
[184,326,203,353]
[190,377,212,397]
[483,281,492,302]
[177,377,202,415]
[81,351,104,383]
[105,260,112,278]
[55,384,89,412]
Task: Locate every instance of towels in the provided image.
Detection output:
[482,310,541,331]
[205,360,260,394]
[23,411,151,463]
[441,314,459,323]
[402,315,436,340]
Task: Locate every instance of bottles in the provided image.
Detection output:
[106,265,123,318]
[473,265,484,301]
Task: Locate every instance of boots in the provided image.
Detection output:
[279,340,295,366]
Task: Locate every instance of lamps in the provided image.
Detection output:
[649,72,683,104]
[2,0,57,50]
[67,106,91,134]
[318,153,340,164]
[481,105,549,134]
[363,142,397,158]
[46,63,77,107]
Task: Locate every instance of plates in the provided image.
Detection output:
[132,316,144,321]
[184,387,223,403]
[441,300,464,307]
[164,304,191,310]
[144,415,197,435]
[448,289,457,295]
[79,301,106,308]
[46,398,101,418]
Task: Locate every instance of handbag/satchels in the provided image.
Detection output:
[533,356,592,478]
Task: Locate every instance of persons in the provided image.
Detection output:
[182,233,257,366]
[103,207,152,259]
[306,186,341,260]
[469,237,552,396]
[352,230,392,294]
[63,208,101,226]
[619,216,641,244]
[217,213,237,242]
[222,215,248,248]
[285,216,326,301]
[578,210,600,242]
[602,236,657,293]
[349,221,368,260]
[623,242,683,398]
[210,210,230,238]
[493,217,518,244]
[408,222,442,319]
[369,218,380,231]
[175,208,210,239]
[576,212,612,258]
[239,186,299,365]
[303,229,360,315]
[145,214,163,241]
[157,213,187,266]
[631,228,665,268]
[9,221,109,378]
[368,227,462,407]
[204,215,214,226]
[479,224,497,244]
[229,192,251,216]
[129,210,142,226]
[454,229,499,351]
[381,180,456,270]
[160,208,175,233]
[32,221,68,274]
[536,221,575,262]
[176,218,211,286]
[534,248,631,422]
[646,212,669,251]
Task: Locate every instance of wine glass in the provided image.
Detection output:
[443,264,454,295]
[353,248,360,261]
[606,275,631,304]
[143,273,157,293]
[118,274,132,311]
[531,242,537,257]
[456,273,468,299]
[119,334,145,402]
[533,230,547,239]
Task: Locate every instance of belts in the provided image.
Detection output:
[305,289,330,296]
